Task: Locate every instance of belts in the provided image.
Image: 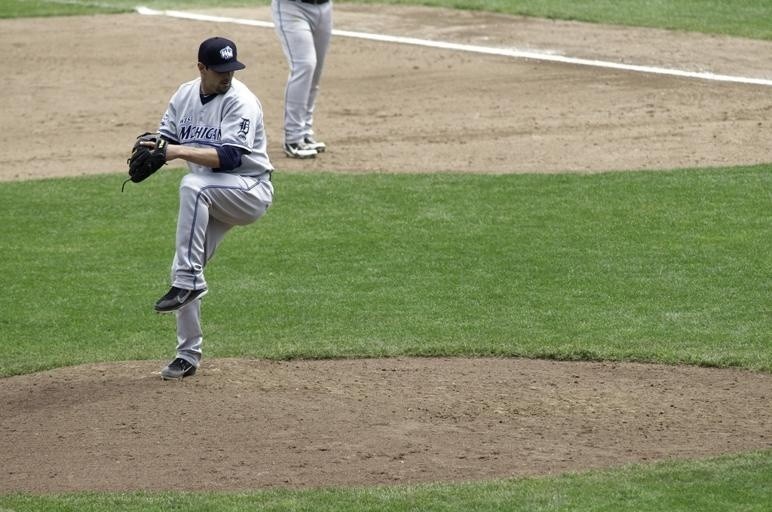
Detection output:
[301,0,330,5]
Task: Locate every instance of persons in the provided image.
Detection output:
[269,0,332,159]
[128,35,276,382]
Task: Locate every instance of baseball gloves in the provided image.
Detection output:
[122,132,167,192]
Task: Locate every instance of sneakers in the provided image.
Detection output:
[284,136,326,159]
[154,284,209,313]
[160,357,197,380]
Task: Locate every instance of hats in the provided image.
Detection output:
[198,37,246,73]
